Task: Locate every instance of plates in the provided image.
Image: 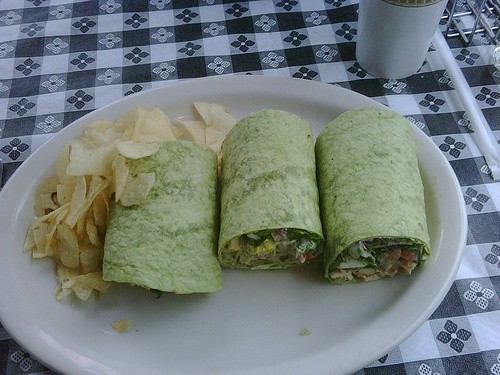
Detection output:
[0,78,468,375]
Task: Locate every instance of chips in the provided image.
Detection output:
[21,102,237,304]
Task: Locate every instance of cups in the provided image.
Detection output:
[356,0,448,79]
